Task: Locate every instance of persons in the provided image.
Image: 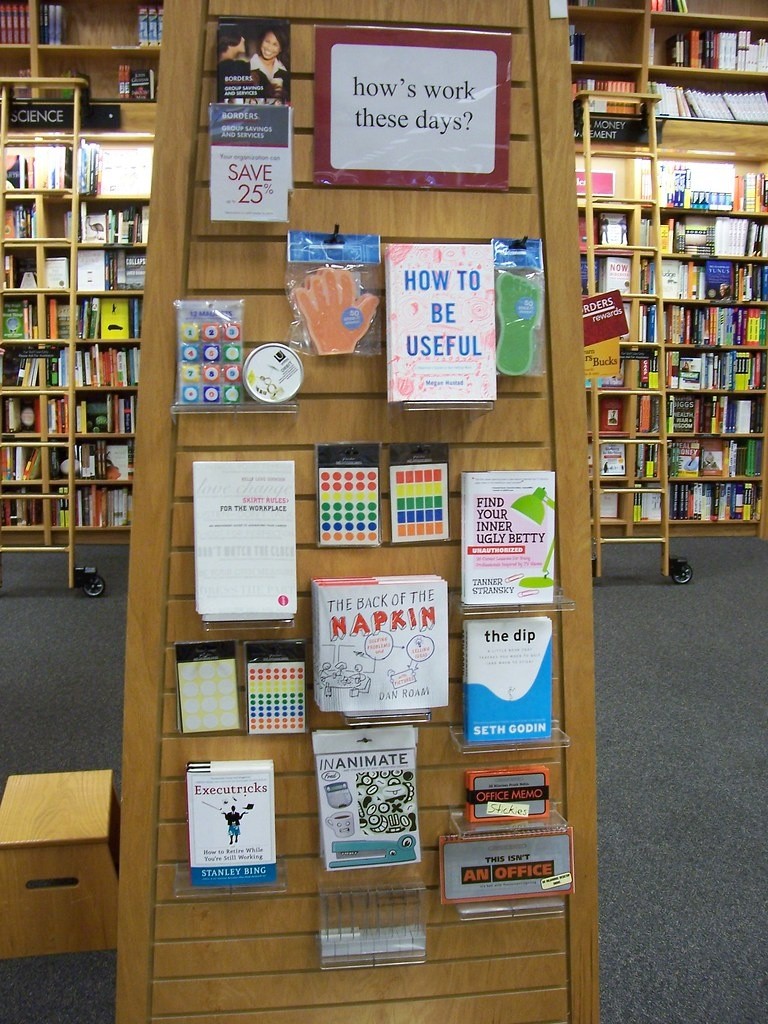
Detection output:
[218,25,287,106]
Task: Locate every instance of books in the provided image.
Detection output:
[0,0,164,528]
[462,616,552,745]
[310,574,450,712]
[193,460,298,614]
[385,245,498,403]
[460,471,556,606]
[217,15,292,108]
[186,759,278,888]
[568,0,768,522]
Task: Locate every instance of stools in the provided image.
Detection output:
[0,769,119,958]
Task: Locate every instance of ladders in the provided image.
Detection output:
[0,77,107,598]
[573,88,693,586]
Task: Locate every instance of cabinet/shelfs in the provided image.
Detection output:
[565,0,768,538]
[0,0,170,547]
